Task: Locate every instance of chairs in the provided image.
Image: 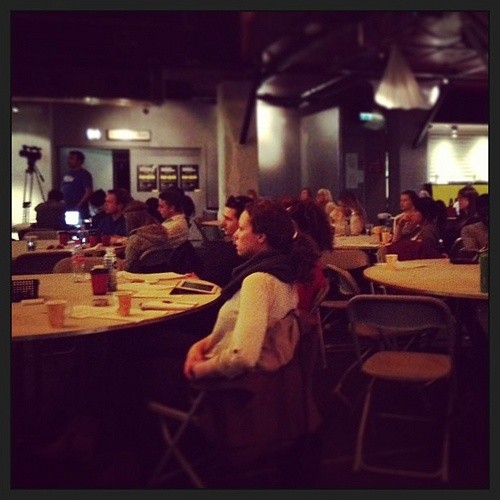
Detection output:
[10,201,469,483]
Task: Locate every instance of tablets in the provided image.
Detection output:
[175,279,218,293]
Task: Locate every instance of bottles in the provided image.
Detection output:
[103,247,117,291]
[480,249,489,293]
[72,245,84,282]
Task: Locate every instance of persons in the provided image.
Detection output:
[53,151,93,218]
[35,184,489,491]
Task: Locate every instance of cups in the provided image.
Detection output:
[25,236,38,252]
[47,300,66,328]
[115,291,133,315]
[385,254,398,271]
[382,232,391,245]
[59,232,68,245]
[102,232,111,247]
[89,232,98,247]
[89,267,109,295]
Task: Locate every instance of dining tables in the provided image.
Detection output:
[10,272,222,489]
[362,255,488,300]
[12,237,121,258]
[333,235,391,249]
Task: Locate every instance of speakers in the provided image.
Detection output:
[404,96,443,150]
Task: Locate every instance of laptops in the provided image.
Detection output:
[65,210,81,228]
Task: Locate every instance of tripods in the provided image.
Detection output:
[23,158,46,222]
